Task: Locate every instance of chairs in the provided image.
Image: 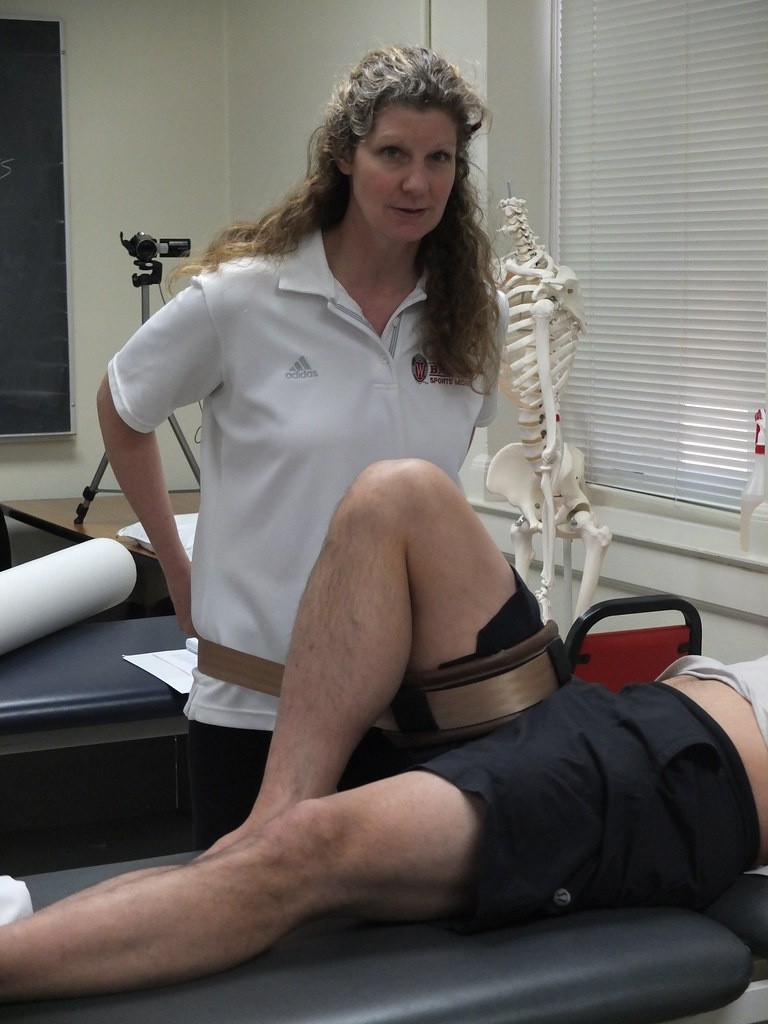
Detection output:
[560,595,702,697]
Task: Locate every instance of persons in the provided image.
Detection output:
[94,45,511,854]
[0,456,768,1001]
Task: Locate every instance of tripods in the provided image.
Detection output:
[75,262,197,524]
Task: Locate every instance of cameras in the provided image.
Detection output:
[120,231,191,262]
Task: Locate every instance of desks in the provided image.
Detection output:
[0,491,210,615]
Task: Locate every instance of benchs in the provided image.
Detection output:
[0,612,202,839]
[0,843,768,1024]
